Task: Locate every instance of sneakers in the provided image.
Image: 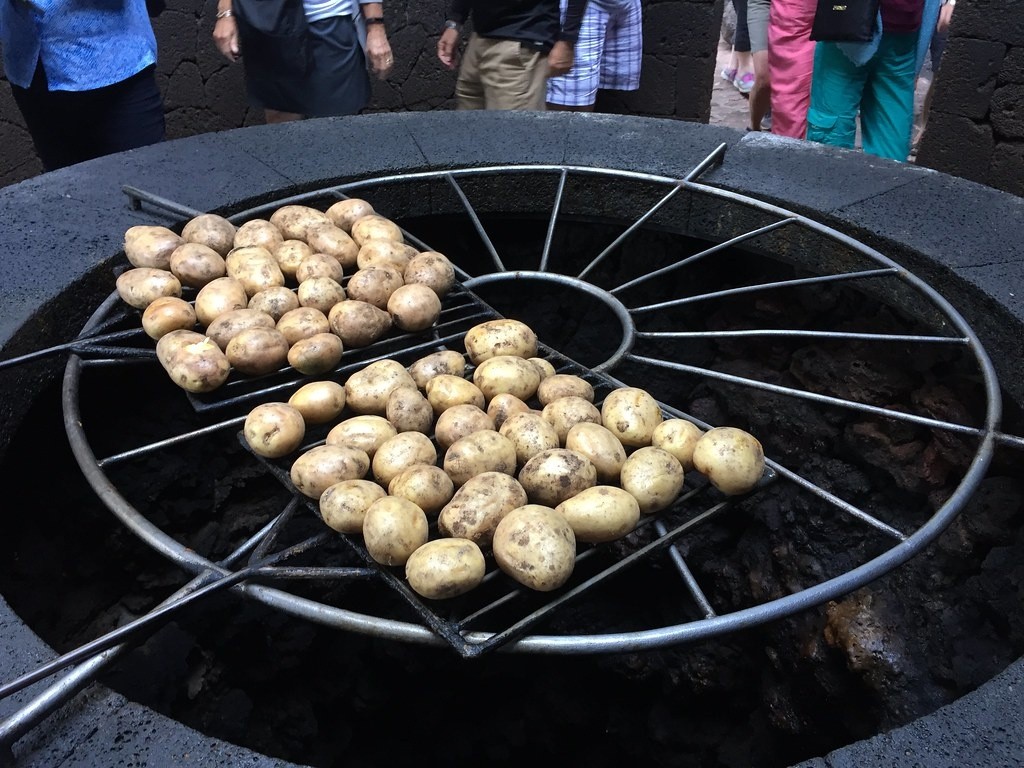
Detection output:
[760,113,772,130]
[721,67,738,83]
[733,72,755,93]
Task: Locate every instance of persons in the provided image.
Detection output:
[546,0,643,114]
[438,0,589,112]
[721,0,755,93]
[914,0,956,98]
[746,0,772,133]
[213,0,393,124]
[0,0,167,172]
[808,0,925,167]
[768,0,818,139]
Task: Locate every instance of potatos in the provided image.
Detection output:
[651,418,701,473]
[437,471,528,547]
[243,320,603,531]
[601,387,664,445]
[619,445,685,514]
[565,422,628,481]
[363,496,428,564]
[492,504,576,592]
[554,485,640,542]
[692,427,765,496]
[405,537,486,600]
[115,197,455,393]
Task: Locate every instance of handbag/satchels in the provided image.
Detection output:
[809,1,880,43]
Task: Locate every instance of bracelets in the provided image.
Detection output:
[216,10,234,18]
[445,21,463,32]
[365,17,384,24]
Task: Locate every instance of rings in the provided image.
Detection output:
[387,60,390,62]
